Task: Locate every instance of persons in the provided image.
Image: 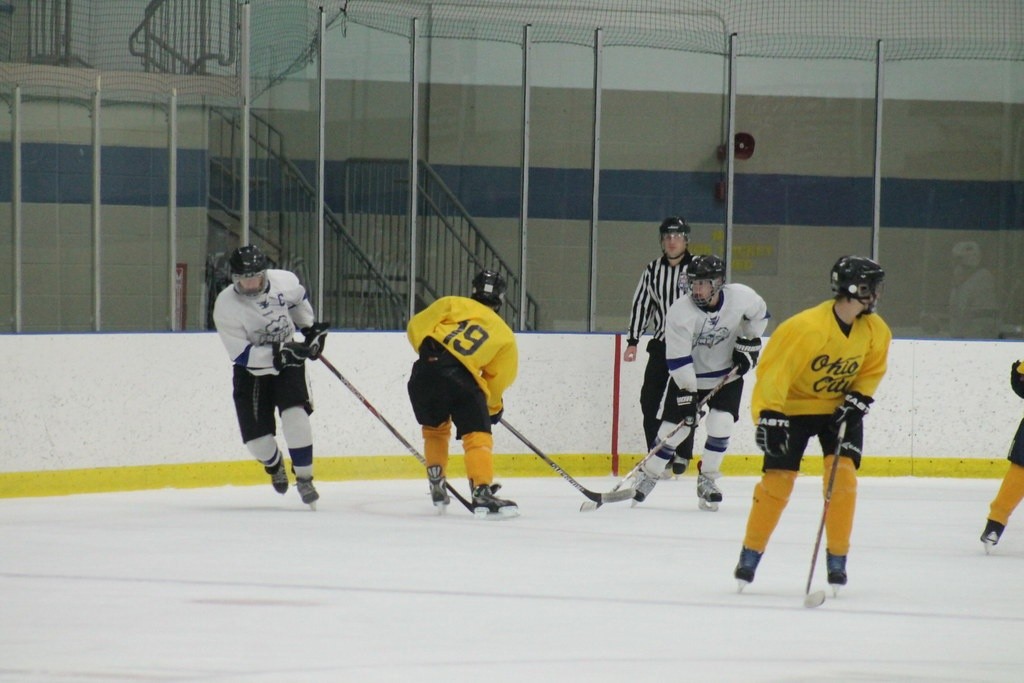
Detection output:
[623,216,702,480]
[980,358,1024,554]
[733,256,893,596]
[213,244,330,514]
[408,271,520,518]
[631,253,769,516]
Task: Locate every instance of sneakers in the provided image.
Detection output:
[265,451,289,495]
[697,461,722,512]
[980,519,1005,555]
[468,478,518,518]
[631,469,660,508]
[826,549,847,597]
[733,546,761,594]
[633,454,690,481]
[291,467,319,511]
[427,464,450,515]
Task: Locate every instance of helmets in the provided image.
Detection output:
[472,269,506,313]
[686,255,725,307]
[831,255,884,315]
[229,245,265,278]
[657,216,691,233]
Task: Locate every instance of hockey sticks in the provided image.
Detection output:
[580,363,742,512]
[803,420,847,610]
[498,418,636,504]
[318,354,501,514]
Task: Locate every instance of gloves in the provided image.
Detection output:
[676,388,706,426]
[832,396,872,435]
[755,408,791,458]
[271,322,327,371]
[732,335,761,374]
[490,408,504,426]
